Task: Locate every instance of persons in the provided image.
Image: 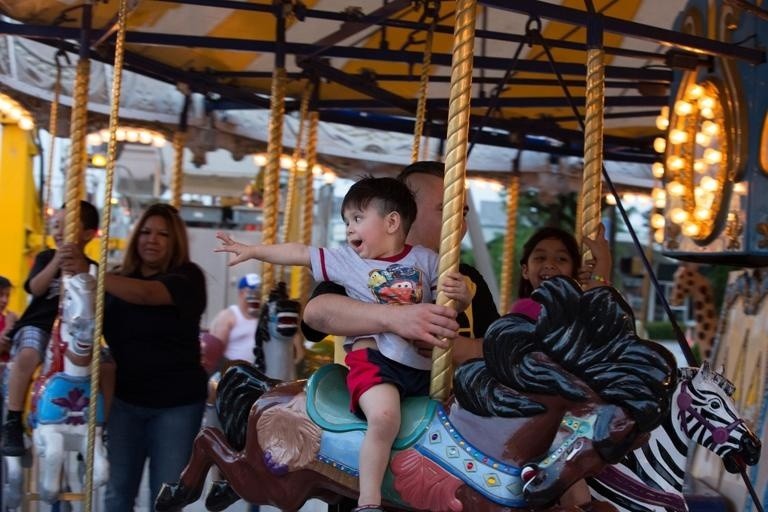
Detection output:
[0,199,100,456]
[506,221,613,323]
[210,273,263,369]
[212,177,475,512]
[100,203,208,512]
[301,161,500,365]
[0,273,19,371]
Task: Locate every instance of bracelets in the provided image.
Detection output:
[590,273,611,286]
[88,263,98,278]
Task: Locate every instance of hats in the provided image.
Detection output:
[238,273,262,289]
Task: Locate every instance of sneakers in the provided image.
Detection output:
[0,419,27,458]
